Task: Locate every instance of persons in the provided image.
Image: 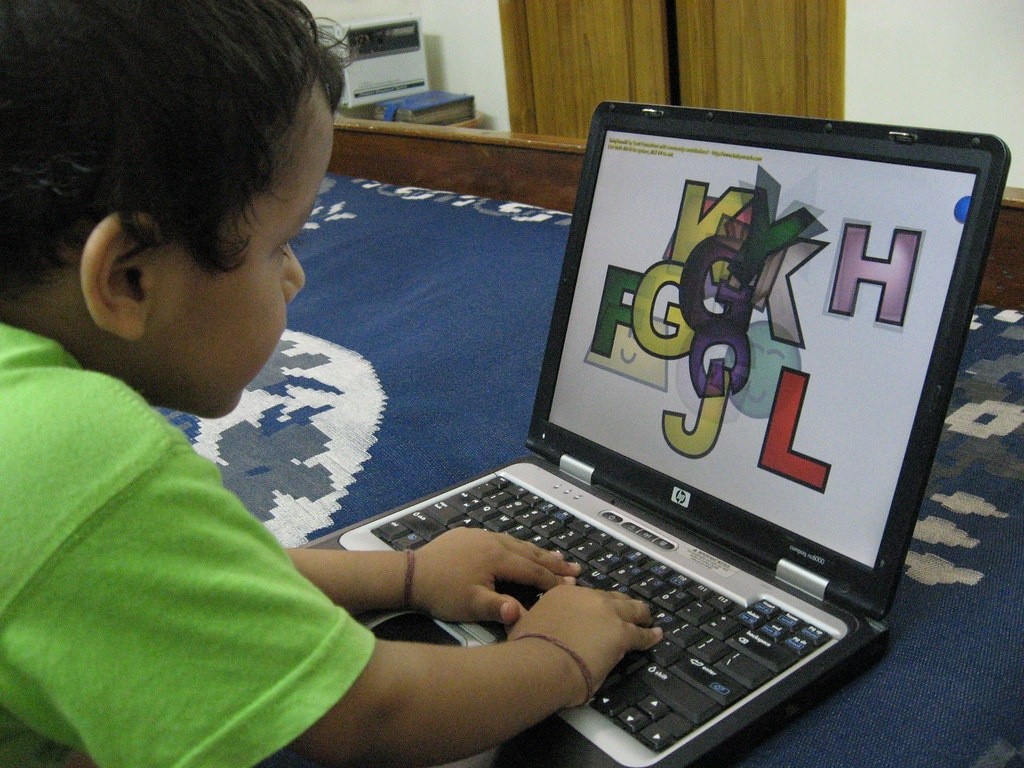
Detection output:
[0,0,661,768]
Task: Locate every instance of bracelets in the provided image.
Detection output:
[403,549,415,610]
[514,631,594,707]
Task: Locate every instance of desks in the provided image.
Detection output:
[150,171,1024,767]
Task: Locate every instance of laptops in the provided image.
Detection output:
[302,101,1012,768]
